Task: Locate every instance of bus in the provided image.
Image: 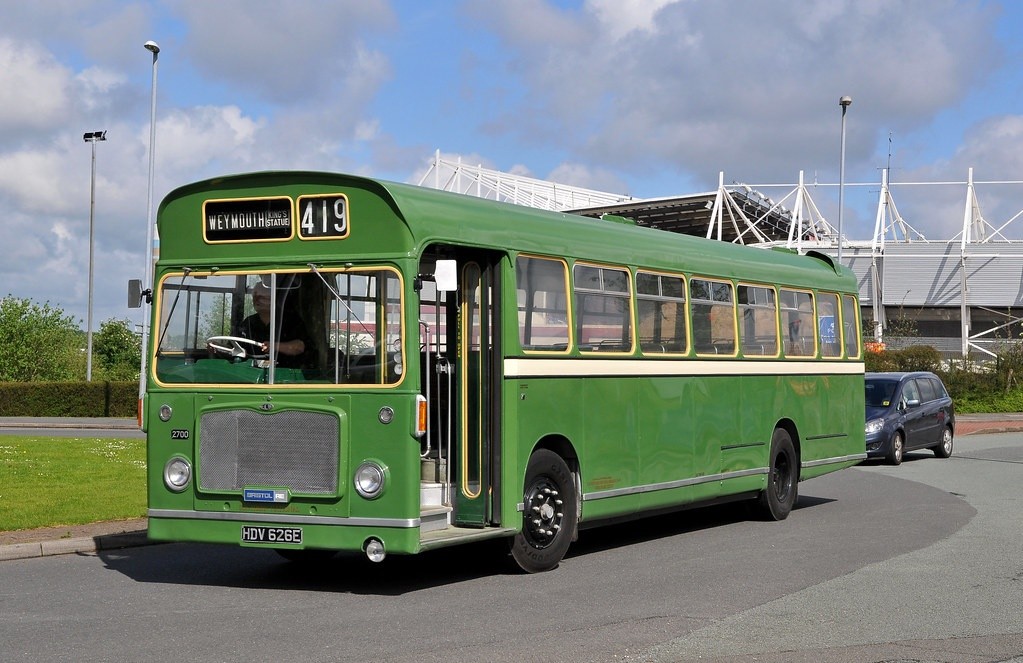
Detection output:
[142,169,868,574]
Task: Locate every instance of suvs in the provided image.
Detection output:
[864,371,956,466]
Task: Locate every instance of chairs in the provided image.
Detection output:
[557,335,837,350]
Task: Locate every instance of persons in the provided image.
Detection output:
[206,280,314,369]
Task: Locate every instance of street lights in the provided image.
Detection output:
[79,129,111,381]
[141,39,162,434]
[836,93,853,264]
